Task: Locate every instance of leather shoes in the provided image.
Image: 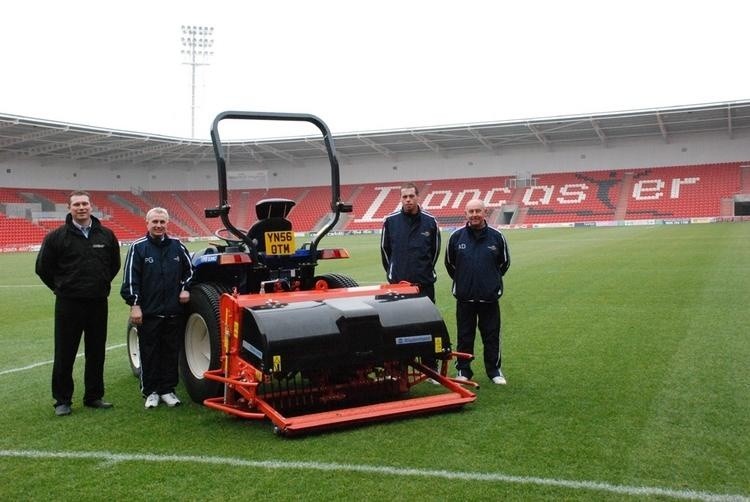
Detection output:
[55,405,70,415]
[84,400,112,408]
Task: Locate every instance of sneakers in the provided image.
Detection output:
[456,376,468,381]
[492,376,506,385]
[425,378,440,385]
[161,392,181,407]
[144,392,161,408]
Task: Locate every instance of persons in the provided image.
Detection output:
[34,191,197,416]
[379,182,510,386]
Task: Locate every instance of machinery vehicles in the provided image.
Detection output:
[127,110,480,438]
